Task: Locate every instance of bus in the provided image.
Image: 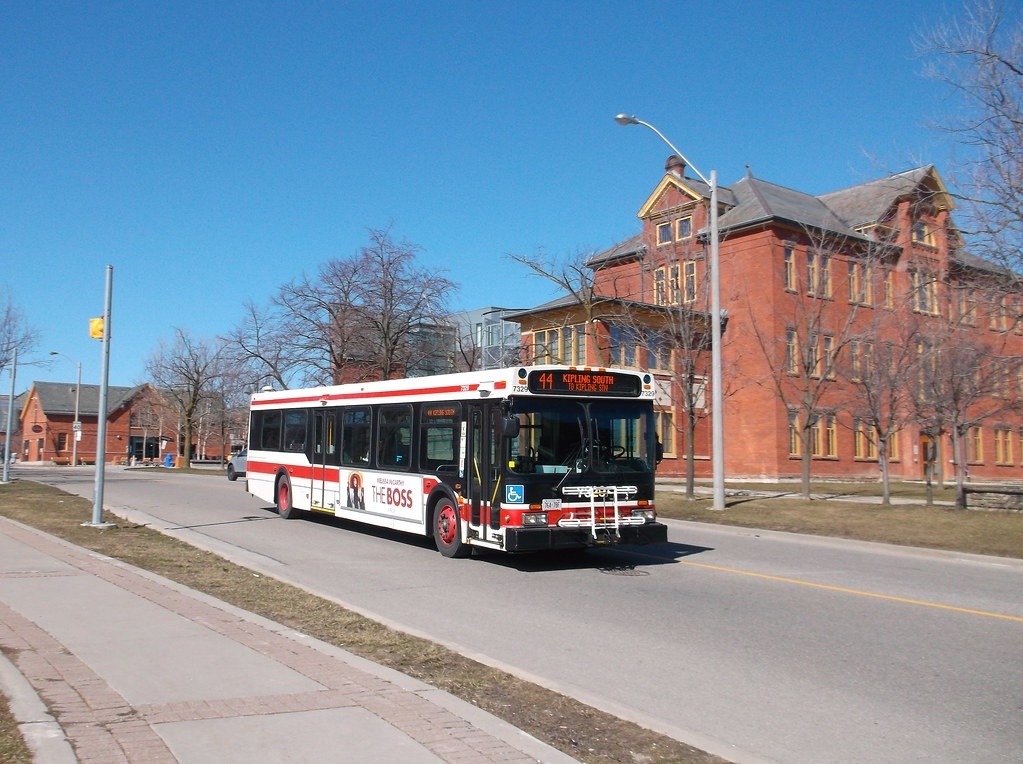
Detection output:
[245,364,668,559]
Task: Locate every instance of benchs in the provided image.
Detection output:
[80,456,96,465]
[137,456,160,468]
[51,457,69,465]
[942,481,1023,512]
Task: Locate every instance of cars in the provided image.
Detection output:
[226,452,239,463]
[226,446,247,480]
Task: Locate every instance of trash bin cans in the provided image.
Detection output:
[120,457,127,465]
[192,454,201,464]
[130,455,137,467]
[144,458,151,466]
[153,458,160,467]
[164,454,172,467]
[10,453,17,464]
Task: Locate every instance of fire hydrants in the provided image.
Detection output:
[131,456,137,466]
[166,454,172,468]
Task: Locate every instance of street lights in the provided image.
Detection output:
[50,352,82,468]
[617,114,725,510]
